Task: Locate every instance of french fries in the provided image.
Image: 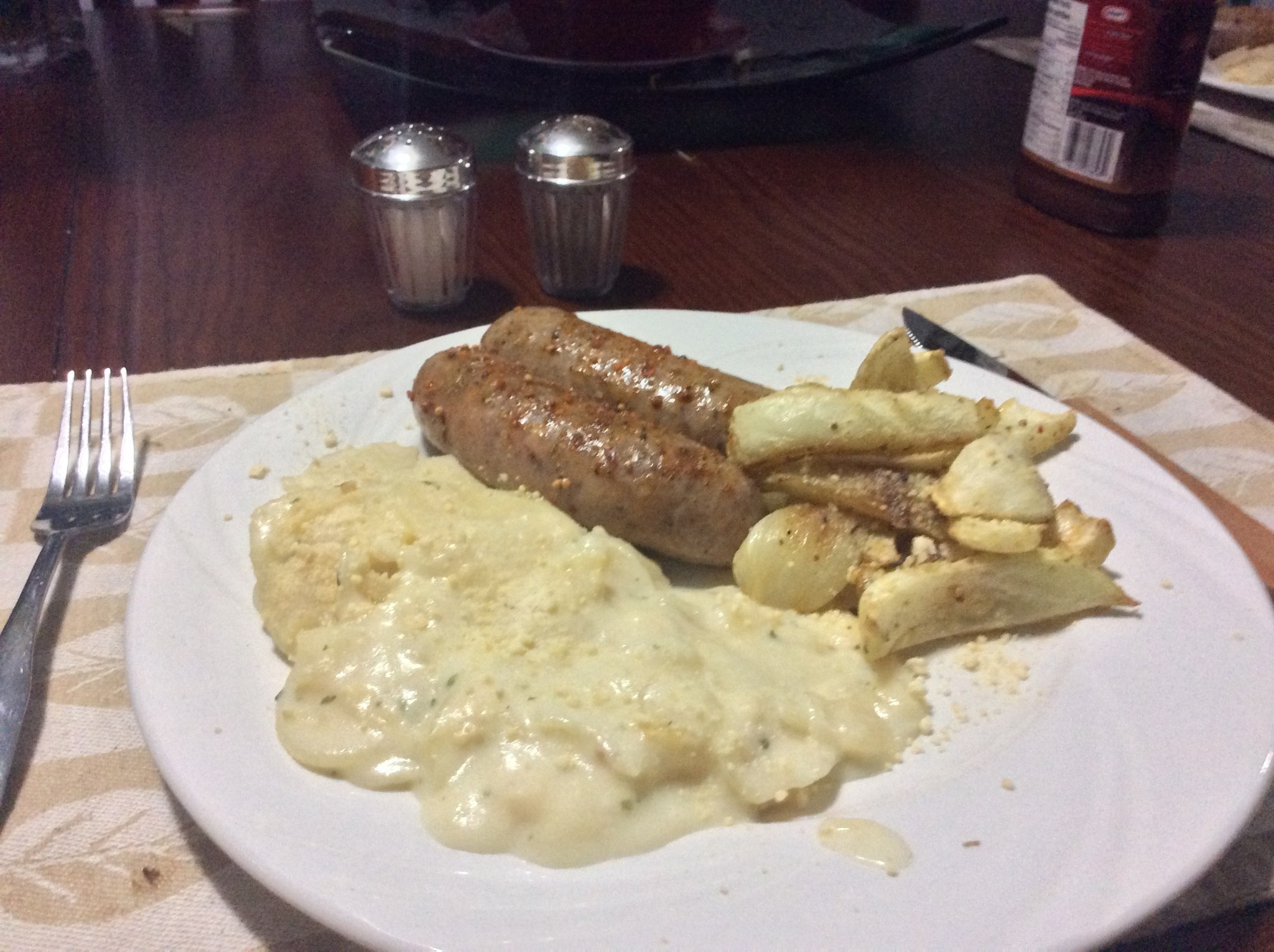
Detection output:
[723,330,1138,659]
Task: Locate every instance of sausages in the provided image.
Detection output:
[408,304,789,567]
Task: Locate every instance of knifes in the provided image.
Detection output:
[898,306,1053,400]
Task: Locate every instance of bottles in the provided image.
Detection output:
[516,114,638,300]
[348,119,478,316]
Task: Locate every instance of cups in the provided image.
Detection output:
[1016,0,1220,236]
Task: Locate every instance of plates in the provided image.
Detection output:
[128,306,1274,952]
[309,0,1011,108]
[1196,68,1274,102]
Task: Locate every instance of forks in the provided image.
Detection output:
[1,366,139,809]
